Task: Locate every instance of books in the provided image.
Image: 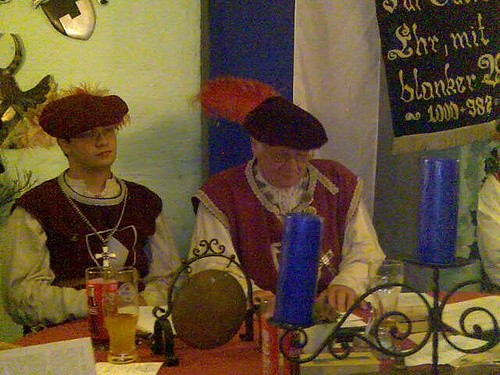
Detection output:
[398,293,443,335]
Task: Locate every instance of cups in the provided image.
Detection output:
[260,295,300,375]
[85,266,119,350]
[102,268,139,365]
[366,259,404,359]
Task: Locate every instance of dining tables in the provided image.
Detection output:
[4,289,500,375]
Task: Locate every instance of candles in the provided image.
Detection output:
[418,153,461,264]
[269,211,322,325]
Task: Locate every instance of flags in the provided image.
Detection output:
[376,0,500,156]
[204,1,383,228]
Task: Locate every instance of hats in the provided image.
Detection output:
[40,91,129,142]
[242,96,328,152]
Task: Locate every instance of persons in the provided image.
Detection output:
[0,78,187,335]
[476,123,499,289]
[183,96,389,320]
